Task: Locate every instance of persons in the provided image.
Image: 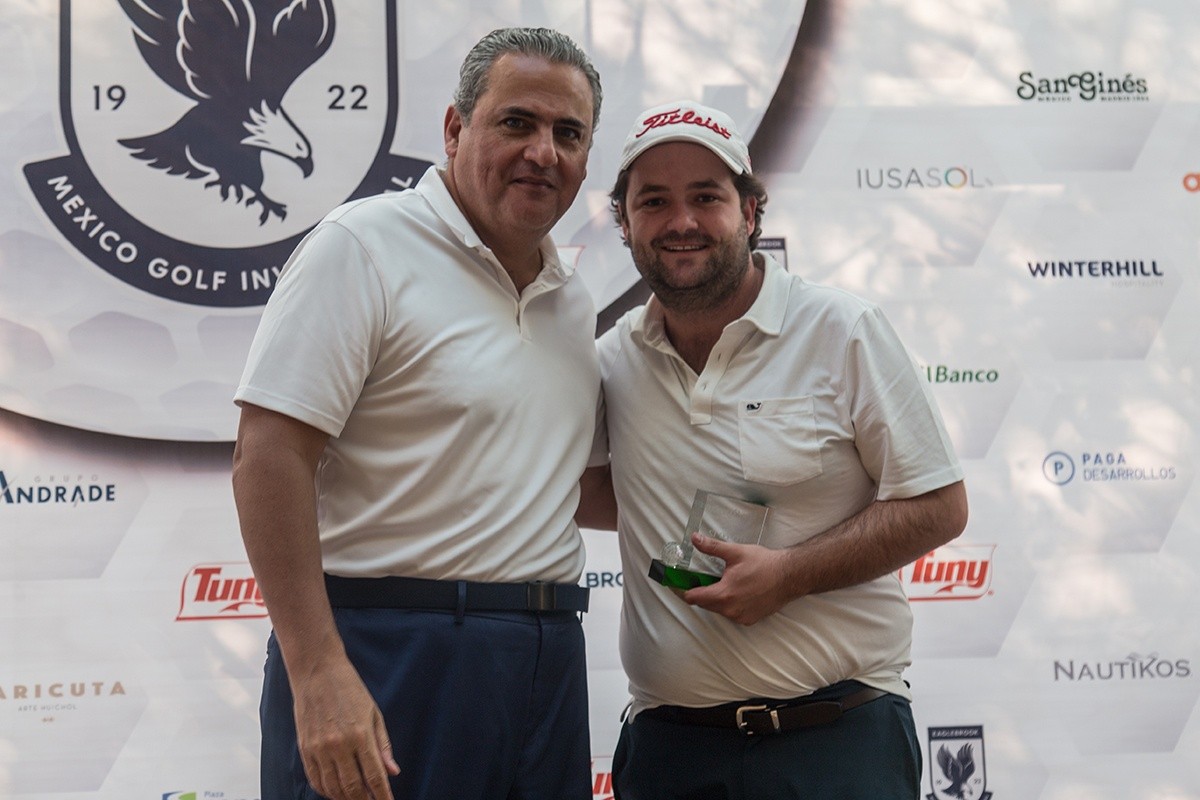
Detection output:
[577,101,968,800]
[232,27,617,800]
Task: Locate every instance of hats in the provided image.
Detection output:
[617,100,753,176]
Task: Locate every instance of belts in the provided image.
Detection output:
[640,687,889,735]
[323,573,589,613]
